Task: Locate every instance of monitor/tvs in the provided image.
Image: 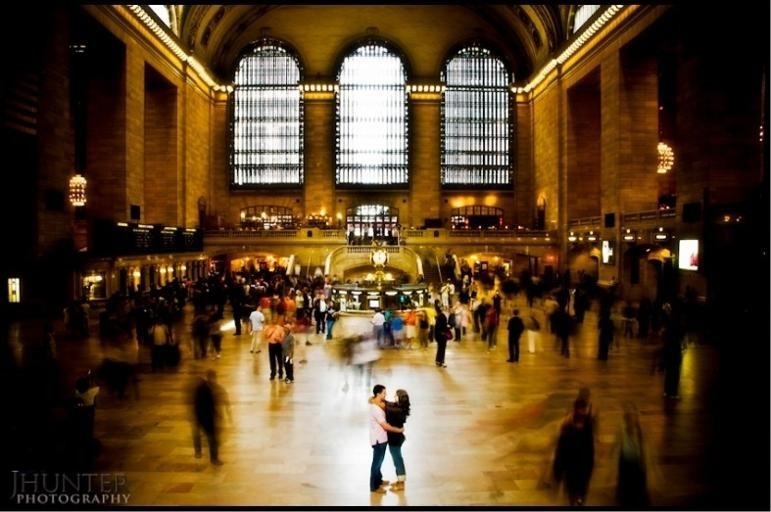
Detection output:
[678,238,701,273]
[601,240,615,264]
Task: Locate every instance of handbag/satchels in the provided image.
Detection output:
[445,331,453,338]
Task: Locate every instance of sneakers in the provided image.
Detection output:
[376,481,404,493]
[269,373,294,383]
[438,362,447,367]
[193,349,221,360]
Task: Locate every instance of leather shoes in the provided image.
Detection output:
[211,458,223,465]
